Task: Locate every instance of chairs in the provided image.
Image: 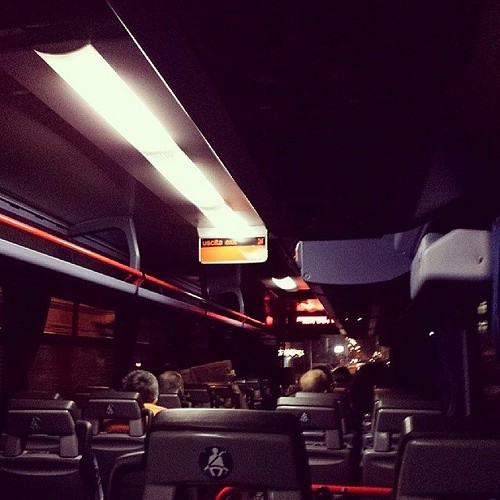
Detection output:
[0,378,500,500]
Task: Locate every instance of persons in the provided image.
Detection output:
[298,361,393,404]
[120,370,191,424]
